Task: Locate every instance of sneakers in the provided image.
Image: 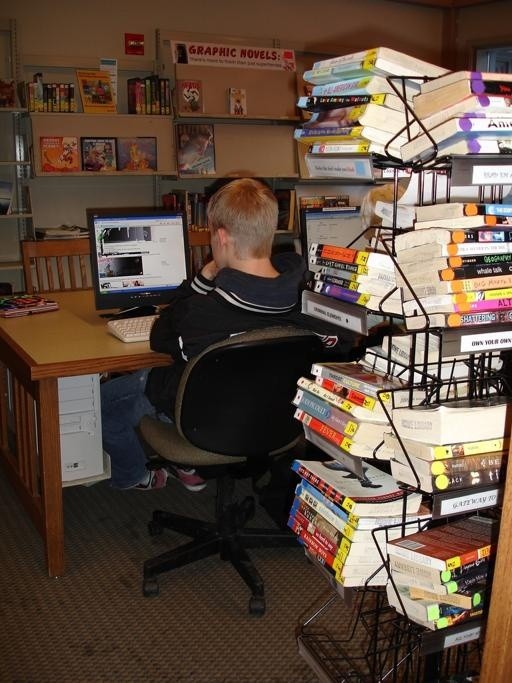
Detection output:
[137,469,170,490]
[172,468,204,491]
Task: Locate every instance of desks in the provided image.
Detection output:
[0,291,174,578]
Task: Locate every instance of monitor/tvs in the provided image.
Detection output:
[85,207,192,318]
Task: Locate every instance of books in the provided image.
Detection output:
[117,137,157,172]
[292,46,454,181]
[28,80,75,113]
[301,243,403,336]
[386,514,499,631]
[1,292,59,318]
[362,331,503,384]
[1,77,15,108]
[172,122,216,176]
[389,395,510,495]
[163,188,210,228]
[227,86,247,117]
[81,135,117,171]
[39,136,80,172]
[74,67,117,113]
[400,70,512,171]
[392,202,511,331]
[128,76,172,115]
[174,78,206,116]
[370,172,446,251]
[0,178,13,213]
[276,187,298,233]
[286,460,431,587]
[293,363,413,460]
[35,223,90,241]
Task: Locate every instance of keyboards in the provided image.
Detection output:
[107,314,160,343]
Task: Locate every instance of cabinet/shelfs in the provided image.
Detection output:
[15,69,297,240]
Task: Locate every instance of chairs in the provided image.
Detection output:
[22,239,96,294]
[144,327,325,615]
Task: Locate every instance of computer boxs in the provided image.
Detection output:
[58,374,104,482]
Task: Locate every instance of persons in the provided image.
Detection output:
[100,178,306,491]
